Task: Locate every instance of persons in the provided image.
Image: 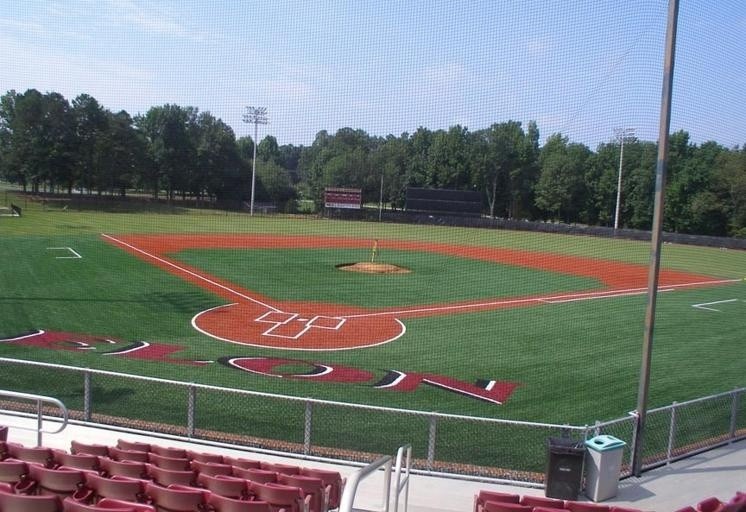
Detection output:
[388,193,397,211]
[370,239,380,263]
[398,191,407,211]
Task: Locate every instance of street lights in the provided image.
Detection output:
[611,126,637,229]
[239,103,271,218]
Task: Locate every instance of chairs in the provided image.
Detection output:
[474,487,745,511]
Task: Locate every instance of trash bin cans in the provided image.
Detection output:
[584,434,627,502]
[545,437,586,501]
